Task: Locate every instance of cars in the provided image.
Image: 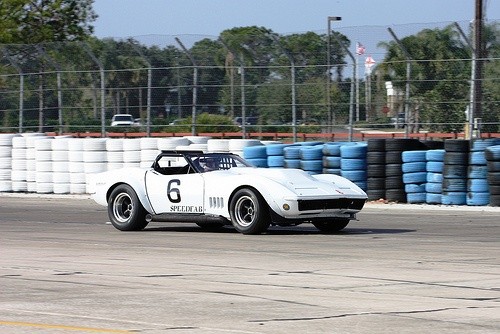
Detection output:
[111,114,134,127]
[391,113,421,128]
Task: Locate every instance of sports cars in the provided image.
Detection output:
[90,150,368,235]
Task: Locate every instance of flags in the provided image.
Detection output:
[364,56,376,68]
[357,42,366,56]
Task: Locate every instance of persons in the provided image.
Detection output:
[198,157,214,173]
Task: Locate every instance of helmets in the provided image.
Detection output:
[199,152,221,171]
